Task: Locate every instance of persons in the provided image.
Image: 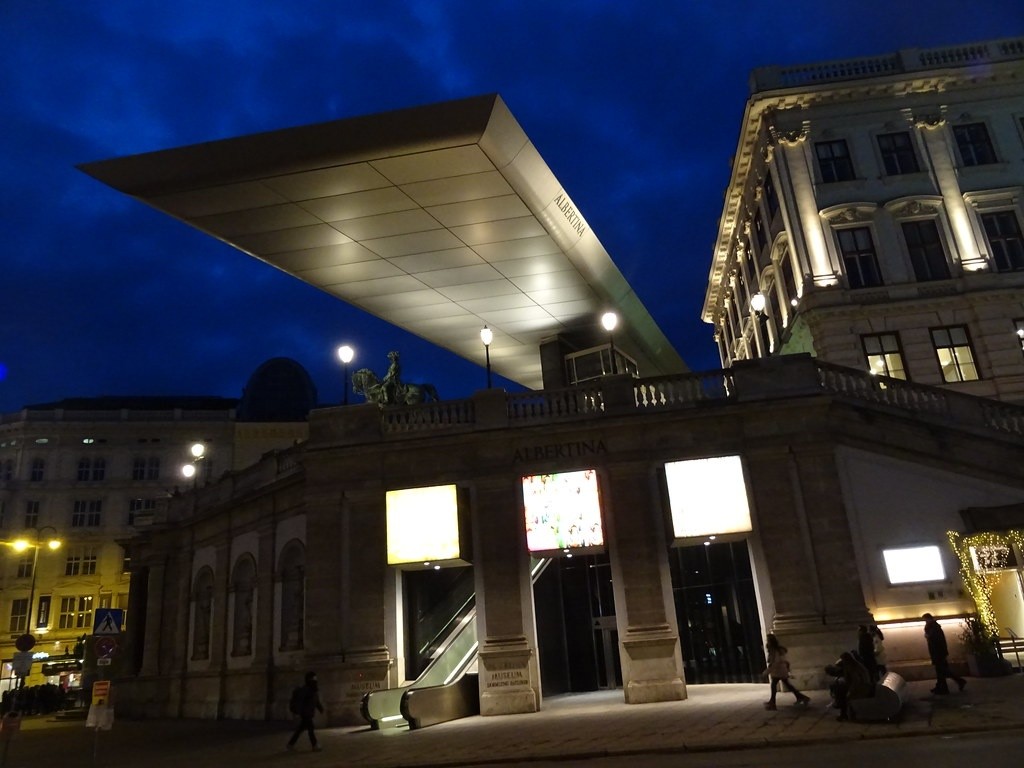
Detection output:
[922,613,966,694]
[379,349,402,405]
[284,671,326,755]
[823,623,889,723]
[761,634,811,707]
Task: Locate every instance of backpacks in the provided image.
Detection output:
[288,682,308,715]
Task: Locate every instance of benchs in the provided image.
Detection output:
[852,671,908,723]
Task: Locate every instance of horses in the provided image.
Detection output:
[351,368,441,407]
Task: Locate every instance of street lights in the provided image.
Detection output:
[191,444,204,491]
[749,292,772,358]
[601,311,619,374]
[338,345,354,406]
[18,525,61,687]
[480,324,493,389]
[183,464,195,491]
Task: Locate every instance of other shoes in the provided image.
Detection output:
[825,701,838,710]
[312,744,323,753]
[286,743,298,750]
[959,677,966,691]
[763,702,777,710]
[931,689,950,697]
[792,695,811,706]
